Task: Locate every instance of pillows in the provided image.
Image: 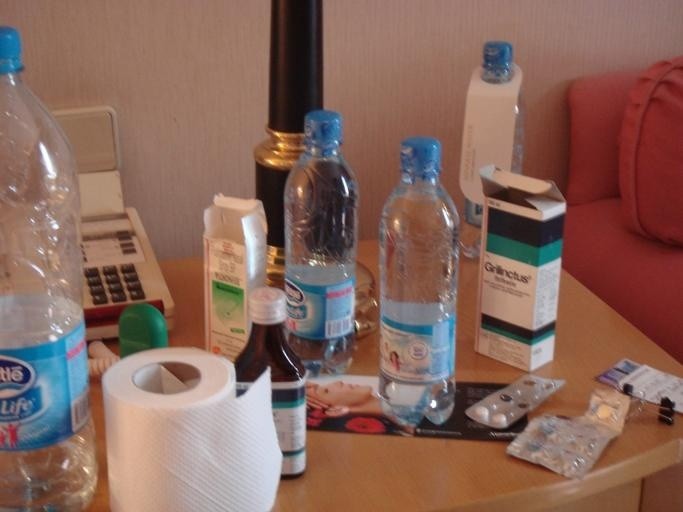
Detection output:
[618,55,683,246]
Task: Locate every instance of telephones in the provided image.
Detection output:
[6,171,175,340]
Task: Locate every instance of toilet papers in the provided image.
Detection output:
[101,347,283,512]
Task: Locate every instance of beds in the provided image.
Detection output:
[558,68,683,370]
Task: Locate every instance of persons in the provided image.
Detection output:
[306,381,381,418]
[389,351,402,372]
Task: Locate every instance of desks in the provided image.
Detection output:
[0,221,683,511]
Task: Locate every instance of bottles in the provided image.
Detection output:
[233,287,307,480]
[378,138,460,429]
[280,108,359,382]
[461,40,526,261]
[0,27,101,512]
